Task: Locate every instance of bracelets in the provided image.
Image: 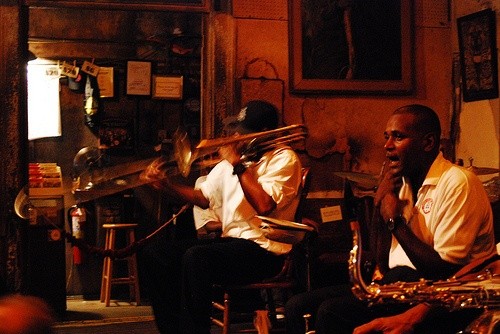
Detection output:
[232,161,249,175]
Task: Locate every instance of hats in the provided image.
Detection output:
[228,99,280,131]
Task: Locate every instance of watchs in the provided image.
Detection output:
[387,217,403,231]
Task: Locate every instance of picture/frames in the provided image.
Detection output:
[287,0,419,96]
[152,73,187,101]
[98,63,118,99]
[456,9,499,103]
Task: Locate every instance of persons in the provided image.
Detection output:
[139,99,301,334]
[0,293,53,334]
[286,104,500,334]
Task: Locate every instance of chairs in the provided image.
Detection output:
[211,168,314,333]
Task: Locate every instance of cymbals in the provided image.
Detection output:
[334,170,386,185]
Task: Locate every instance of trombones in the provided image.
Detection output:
[14,123,309,217]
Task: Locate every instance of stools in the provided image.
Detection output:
[99,222,141,310]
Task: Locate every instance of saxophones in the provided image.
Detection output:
[349,220,500,309]
